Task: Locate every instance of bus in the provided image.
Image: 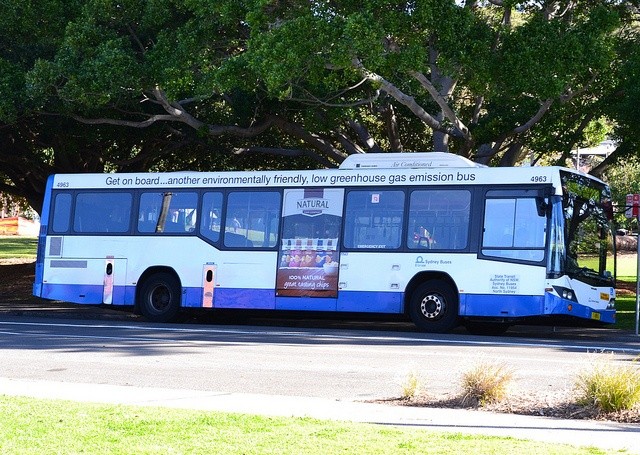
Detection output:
[32,154,618,331]
